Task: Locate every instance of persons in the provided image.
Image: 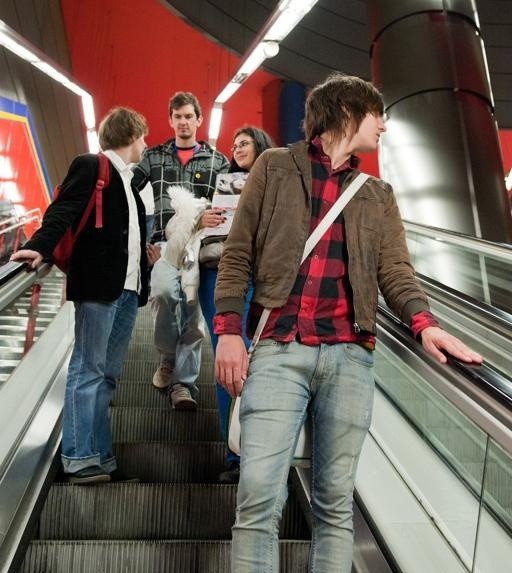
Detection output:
[207,65,488,572]
[130,87,230,411]
[191,124,277,480]
[9,104,162,488]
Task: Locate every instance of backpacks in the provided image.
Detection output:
[52,151,110,274]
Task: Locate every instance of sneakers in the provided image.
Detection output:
[108,469,140,482]
[170,383,198,411]
[152,354,175,388]
[219,462,240,484]
[66,465,111,485]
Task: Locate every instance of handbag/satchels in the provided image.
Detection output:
[227,388,313,467]
